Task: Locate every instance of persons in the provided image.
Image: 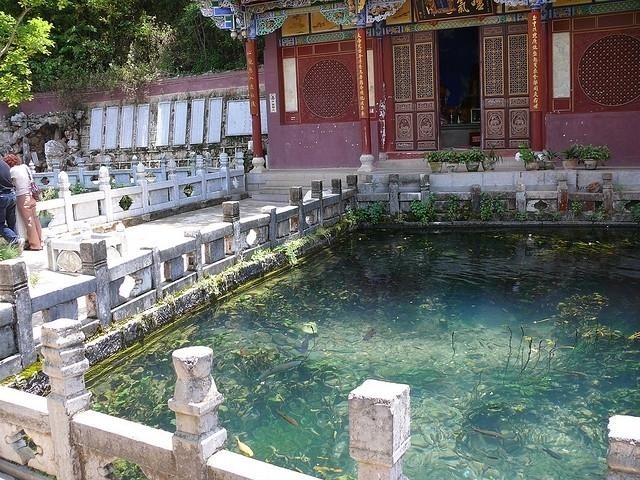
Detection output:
[2,152,42,252]
[0,159,22,251]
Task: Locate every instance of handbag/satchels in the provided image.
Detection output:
[25,166,44,202]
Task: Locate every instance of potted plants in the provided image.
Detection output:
[423,141,611,173]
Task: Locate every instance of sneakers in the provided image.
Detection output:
[23,245,47,250]
